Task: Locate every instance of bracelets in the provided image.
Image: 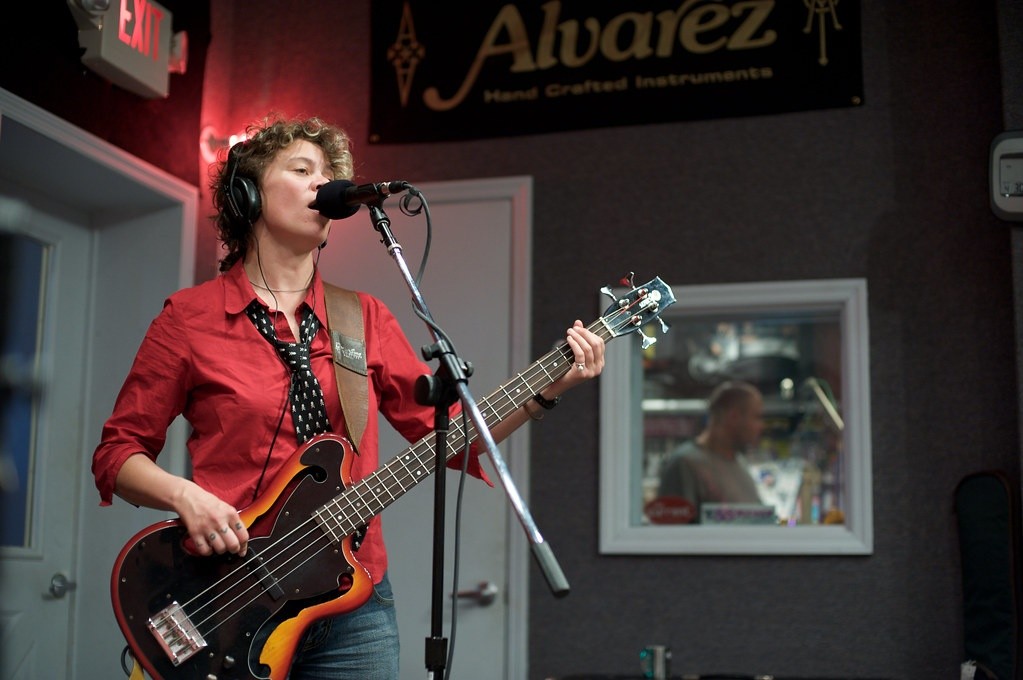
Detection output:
[533,392,561,409]
[523,403,545,421]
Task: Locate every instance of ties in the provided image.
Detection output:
[245,298,368,555]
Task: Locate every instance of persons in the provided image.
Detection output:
[90,110,605,680]
[655,380,845,528]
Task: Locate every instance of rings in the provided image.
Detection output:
[574,361,585,371]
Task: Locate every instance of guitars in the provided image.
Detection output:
[107,268,680,680]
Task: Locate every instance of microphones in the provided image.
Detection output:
[316,179,409,220]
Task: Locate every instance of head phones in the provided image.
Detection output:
[219,140,261,233]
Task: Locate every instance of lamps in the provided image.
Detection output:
[70,0,187,98]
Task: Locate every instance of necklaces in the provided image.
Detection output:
[248,279,310,293]
